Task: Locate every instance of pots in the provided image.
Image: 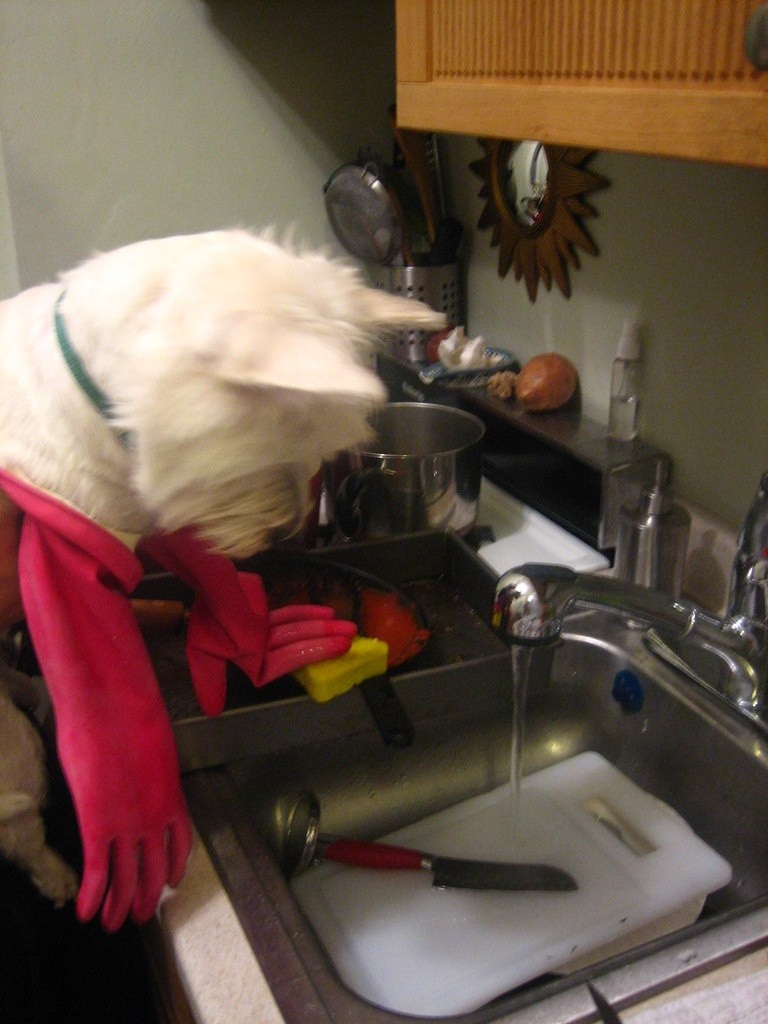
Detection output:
[126,557,430,677]
[325,401,486,542]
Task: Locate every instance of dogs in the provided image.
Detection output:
[0,224,451,918]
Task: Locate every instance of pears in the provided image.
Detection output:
[516,352,577,411]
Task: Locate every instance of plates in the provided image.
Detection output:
[419,346,516,388]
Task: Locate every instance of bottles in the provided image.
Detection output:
[607,321,638,442]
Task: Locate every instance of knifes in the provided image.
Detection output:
[324,838,579,892]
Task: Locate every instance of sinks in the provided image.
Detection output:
[130,524,559,727]
[168,632,768,1024]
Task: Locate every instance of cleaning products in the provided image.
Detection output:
[605,450,693,631]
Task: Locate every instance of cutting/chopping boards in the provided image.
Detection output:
[290,750,732,1018]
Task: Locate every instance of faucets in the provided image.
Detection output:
[489,552,768,735]
